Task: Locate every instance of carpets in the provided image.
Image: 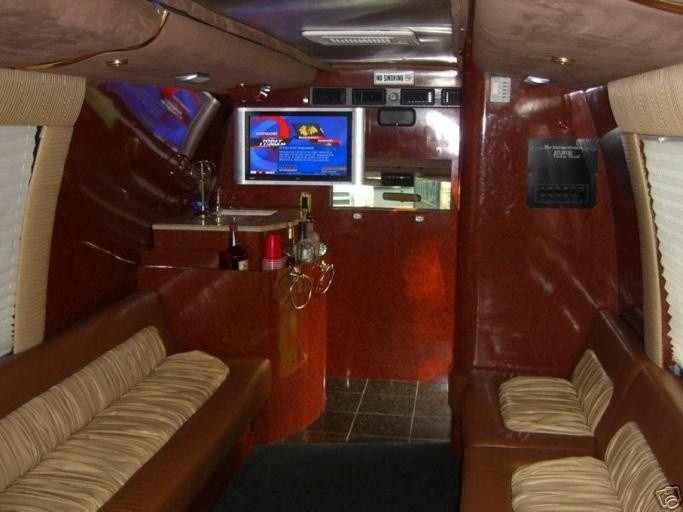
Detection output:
[212,442,458,512]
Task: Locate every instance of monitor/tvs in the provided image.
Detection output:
[233,107,365,188]
[84,81,222,171]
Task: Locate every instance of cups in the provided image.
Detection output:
[263,233,283,271]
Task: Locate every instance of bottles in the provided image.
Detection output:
[297,221,320,263]
[220,223,249,272]
[284,223,303,263]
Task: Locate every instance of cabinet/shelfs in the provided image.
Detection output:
[138,232,330,445]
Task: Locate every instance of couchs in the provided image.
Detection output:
[0,289,273,512]
[459,308,683,512]
